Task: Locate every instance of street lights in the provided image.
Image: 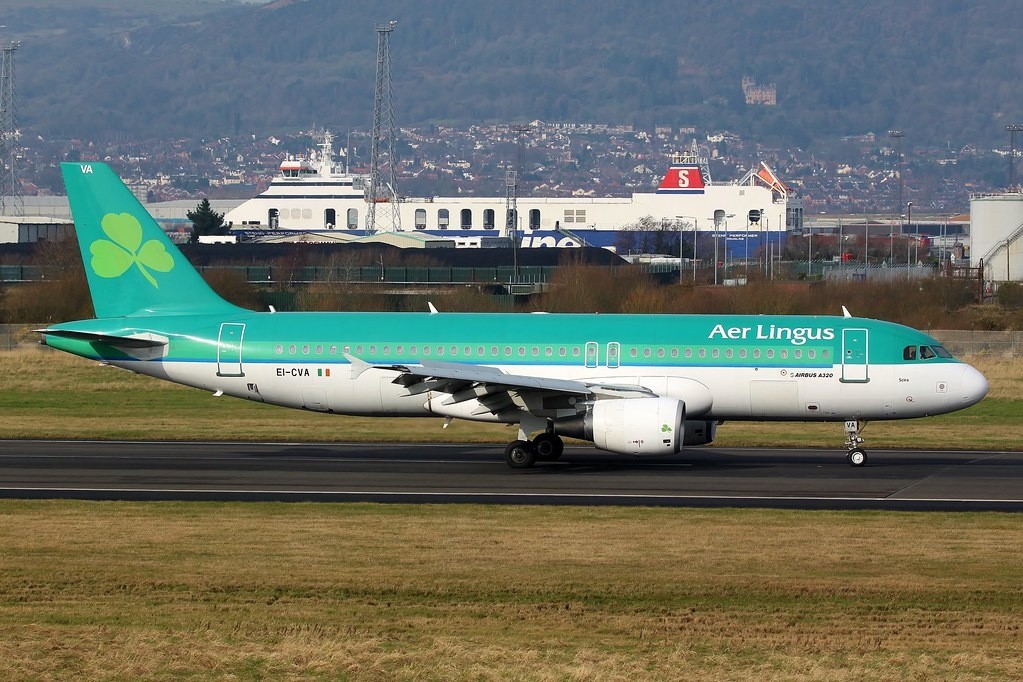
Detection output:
[887,129,906,218]
[1005,125,1023,187]
[900,214,906,236]
[943,215,954,274]
[675,215,697,285]
[907,201,913,284]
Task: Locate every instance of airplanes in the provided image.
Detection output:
[29,161,988,471]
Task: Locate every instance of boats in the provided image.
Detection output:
[221,129,789,263]
